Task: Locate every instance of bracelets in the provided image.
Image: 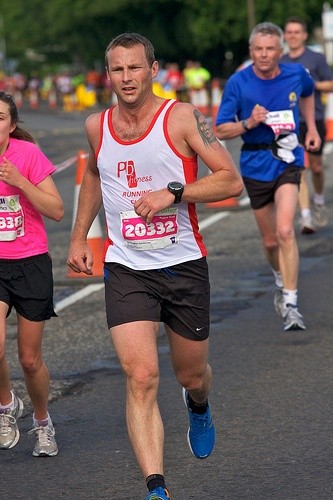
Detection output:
[241,119,249,132]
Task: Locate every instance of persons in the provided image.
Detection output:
[213,20,322,332]
[65,32,246,500]
[278,19,333,235]
[0,59,212,104]
[0,90,66,459]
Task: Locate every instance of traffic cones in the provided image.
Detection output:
[65,151,108,278]
[320,92,333,143]
[13,89,57,111]
[190,78,242,208]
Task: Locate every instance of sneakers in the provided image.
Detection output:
[182,386,216,460]
[27,411,58,456]
[146,487,170,500]
[0,391,24,450]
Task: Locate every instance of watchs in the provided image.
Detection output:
[167,180,185,205]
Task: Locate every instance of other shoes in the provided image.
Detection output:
[273,282,284,318]
[283,305,306,331]
[313,202,329,230]
[299,217,315,234]
